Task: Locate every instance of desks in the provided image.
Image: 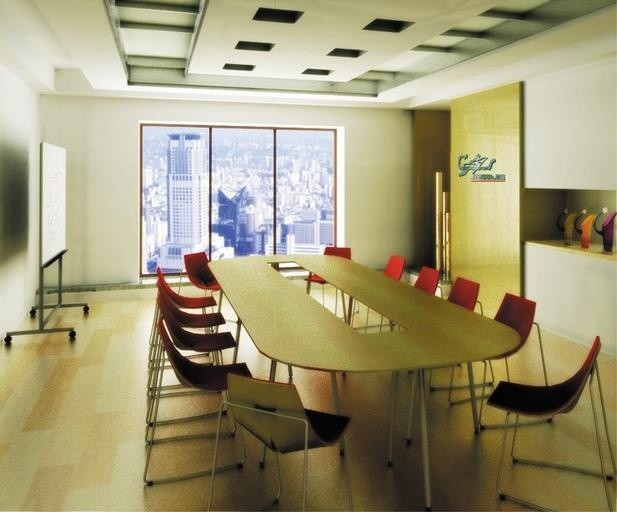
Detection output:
[207,254,521,512]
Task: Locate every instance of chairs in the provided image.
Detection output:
[207,374,353,512]
[148,278,227,369]
[407,276,495,392]
[378,267,443,332]
[449,292,555,430]
[143,318,254,487]
[487,335,617,512]
[351,255,407,335]
[184,251,238,330]
[303,247,360,320]
[148,266,217,362]
[145,293,230,425]
